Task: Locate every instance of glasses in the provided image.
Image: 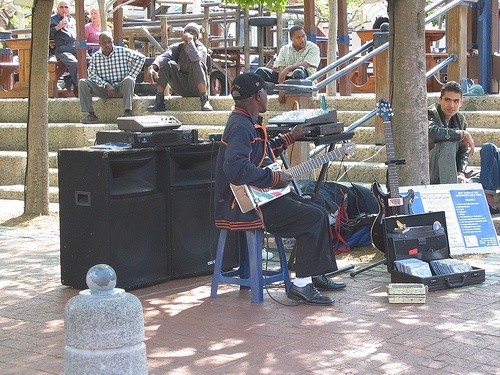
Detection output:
[59,6,68,8]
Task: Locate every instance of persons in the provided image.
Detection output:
[78,31,147,124]
[49,1,78,98]
[428,81,475,185]
[480,143,500,215]
[214,72,346,305]
[147,22,214,112]
[255,26,321,104]
[84,7,112,68]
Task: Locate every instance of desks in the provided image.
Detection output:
[355,28,446,96]
[6,36,69,99]
[210,45,277,76]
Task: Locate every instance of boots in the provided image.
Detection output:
[200,92,214,111]
[147,93,166,111]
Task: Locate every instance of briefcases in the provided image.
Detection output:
[383,211,485,291]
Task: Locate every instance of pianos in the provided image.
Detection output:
[264,108,356,277]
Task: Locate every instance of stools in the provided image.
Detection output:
[206,223,291,304]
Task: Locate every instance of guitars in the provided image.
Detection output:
[230,140,357,214]
[371,98,416,254]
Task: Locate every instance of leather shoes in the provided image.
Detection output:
[288,282,335,305]
[312,274,346,290]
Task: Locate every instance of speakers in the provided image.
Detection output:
[57,141,241,292]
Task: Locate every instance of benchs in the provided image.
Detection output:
[424,51,450,61]
[0,61,21,70]
[48,58,69,68]
[318,54,374,63]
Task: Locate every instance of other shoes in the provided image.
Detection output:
[488,205,500,216]
[122,110,132,116]
[64,75,73,92]
[74,85,78,97]
[80,114,98,123]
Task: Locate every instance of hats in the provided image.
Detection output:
[231,73,267,99]
[463,84,485,95]
[183,21,200,39]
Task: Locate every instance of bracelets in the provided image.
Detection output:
[462,130,466,140]
